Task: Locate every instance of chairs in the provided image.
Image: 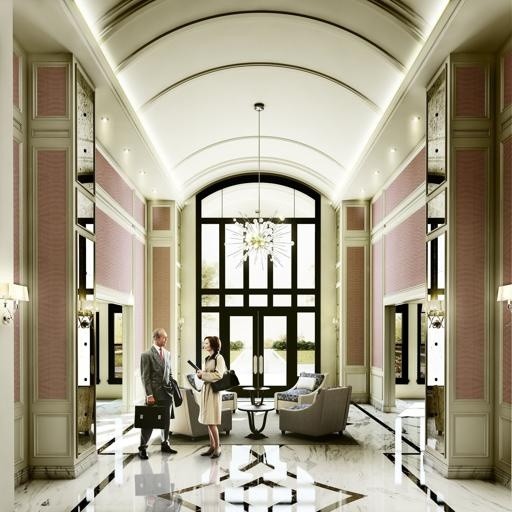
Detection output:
[187,374,237,413]
[274,372,327,415]
[169,388,234,442]
[279,385,352,439]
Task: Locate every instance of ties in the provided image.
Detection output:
[159,347,164,361]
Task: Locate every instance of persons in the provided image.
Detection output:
[186,336,239,459]
[134,328,183,459]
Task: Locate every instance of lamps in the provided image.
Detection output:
[425,299,444,329]
[224,102,295,262]
[496,284,512,309]
[77,300,95,328]
[0,283,29,324]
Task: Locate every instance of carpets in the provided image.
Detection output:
[152,401,360,445]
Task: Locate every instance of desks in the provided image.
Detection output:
[237,404,275,440]
[242,386,271,405]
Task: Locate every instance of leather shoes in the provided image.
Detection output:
[139,448,148,459]
[161,447,177,454]
[210,451,221,460]
[200,448,215,456]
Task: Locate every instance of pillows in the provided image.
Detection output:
[297,377,316,391]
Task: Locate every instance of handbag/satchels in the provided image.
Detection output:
[134,405,171,430]
[211,370,239,393]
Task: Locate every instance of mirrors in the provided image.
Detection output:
[425,230,446,458]
[425,62,448,197]
[425,187,446,238]
[75,62,96,197]
[76,230,97,462]
[76,187,96,236]
